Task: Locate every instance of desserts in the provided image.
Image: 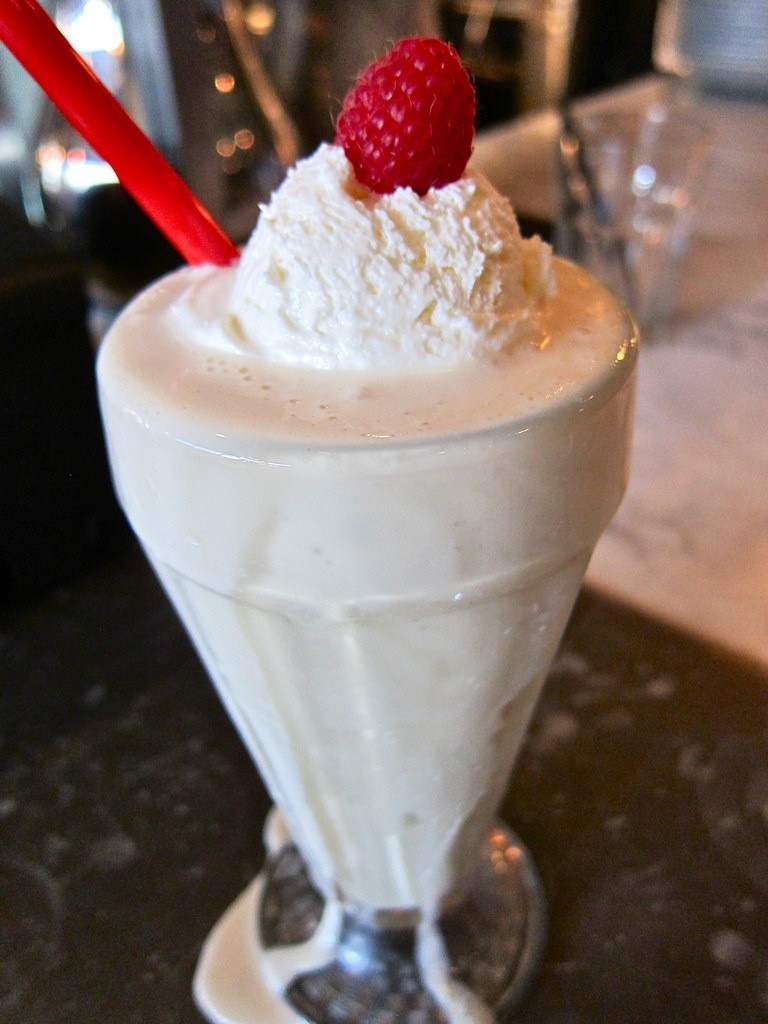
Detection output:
[93,32,641,908]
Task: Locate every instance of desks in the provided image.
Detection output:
[0,73,768,1024]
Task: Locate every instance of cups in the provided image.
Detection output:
[676,0,767,105]
[555,112,707,332]
[98,274,640,1021]
